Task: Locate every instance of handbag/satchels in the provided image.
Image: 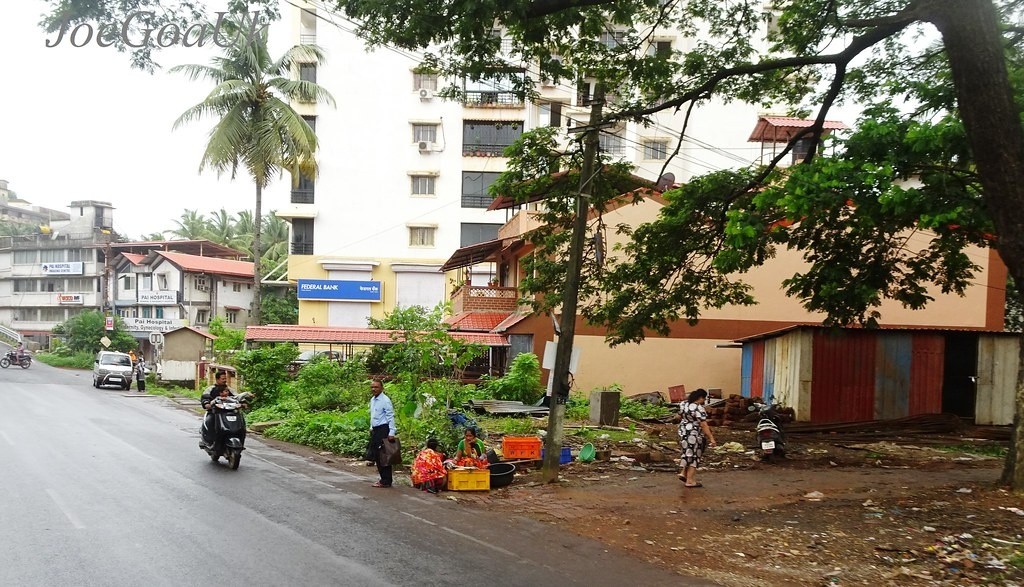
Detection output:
[382,437,402,465]
[366,441,374,461]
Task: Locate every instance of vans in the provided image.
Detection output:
[92,351,135,391]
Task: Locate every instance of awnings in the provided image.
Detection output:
[438,235,518,286]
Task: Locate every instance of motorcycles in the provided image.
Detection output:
[0,348,32,369]
[747,394,787,465]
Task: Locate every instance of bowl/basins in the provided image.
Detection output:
[579,443,596,462]
[488,463,518,488]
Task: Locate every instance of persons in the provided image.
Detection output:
[675,388,716,487]
[129,350,146,392]
[411,438,447,494]
[200,371,247,450]
[454,426,487,462]
[369,379,396,487]
[15,341,25,364]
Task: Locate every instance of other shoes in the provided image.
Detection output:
[686,482,702,487]
[372,481,391,487]
[679,475,686,482]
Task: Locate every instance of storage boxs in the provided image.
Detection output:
[502,436,541,460]
[446,469,492,491]
[541,447,572,464]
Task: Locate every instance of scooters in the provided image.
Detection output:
[199,397,252,470]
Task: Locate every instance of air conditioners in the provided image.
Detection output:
[417,140,432,152]
[418,88,433,99]
